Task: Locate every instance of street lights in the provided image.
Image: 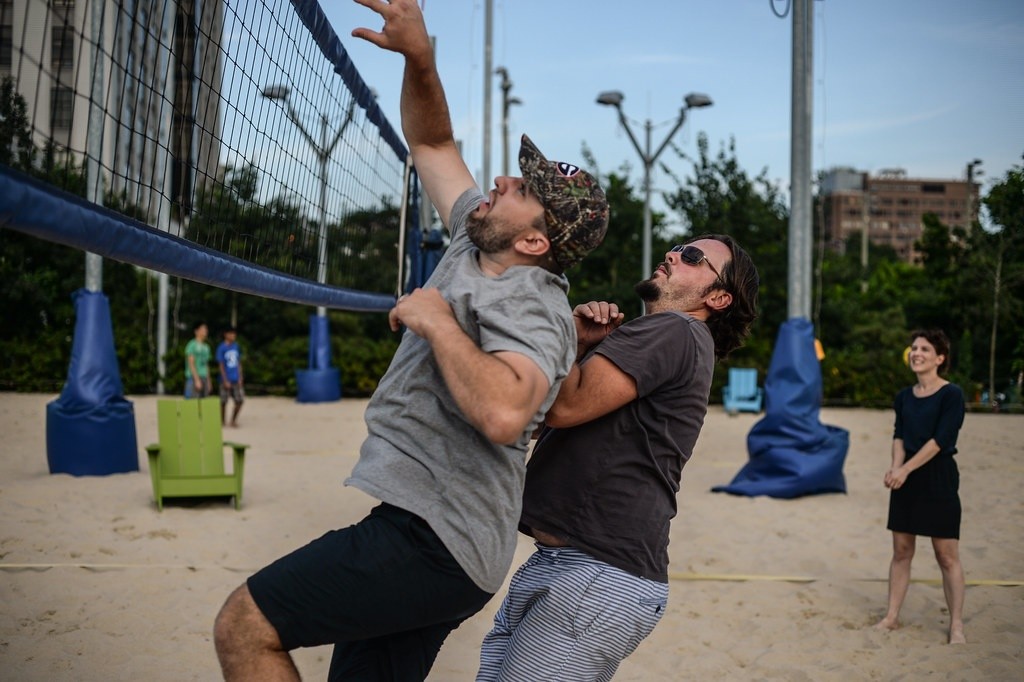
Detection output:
[262,86,378,401]
[596,88,715,315]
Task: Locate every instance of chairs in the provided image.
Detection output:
[721,366,763,414]
[145,397,250,514]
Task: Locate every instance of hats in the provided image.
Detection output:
[518,133,609,271]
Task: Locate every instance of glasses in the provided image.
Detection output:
[670,245,730,292]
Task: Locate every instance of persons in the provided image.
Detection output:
[473,230,761,682]
[216,327,244,428]
[872,329,966,645]
[213,0,613,682]
[184,322,213,398]
